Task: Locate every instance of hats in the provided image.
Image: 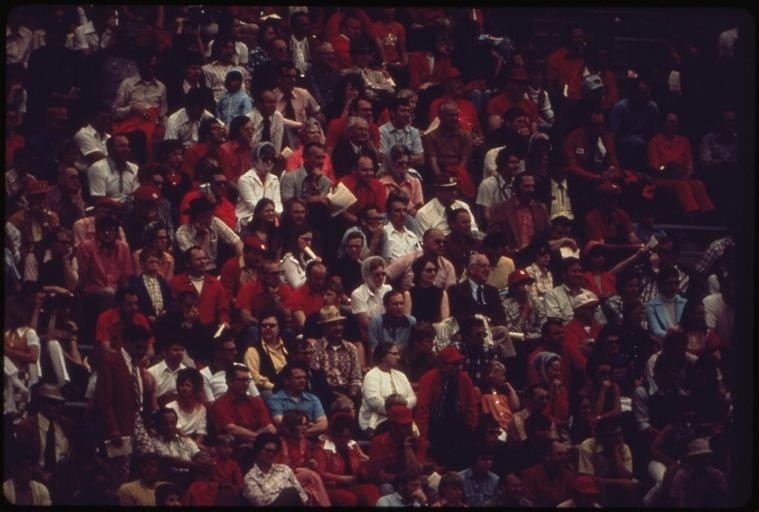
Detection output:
[571,476,598,494]
[317,306,346,325]
[25,181,50,195]
[133,186,161,202]
[439,345,462,362]
[507,268,534,285]
[388,403,412,425]
[181,196,216,214]
[683,439,715,455]
[435,176,459,188]
[572,293,598,312]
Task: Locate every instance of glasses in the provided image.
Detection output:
[261,322,276,330]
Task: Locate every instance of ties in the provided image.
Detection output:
[476,286,483,304]
[45,419,54,461]
[130,362,141,408]
[263,117,271,142]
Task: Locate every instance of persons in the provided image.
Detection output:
[2,1,758,512]
